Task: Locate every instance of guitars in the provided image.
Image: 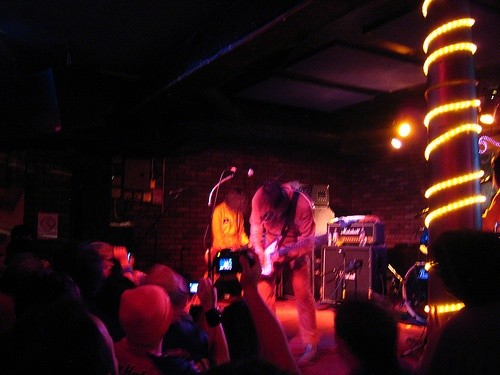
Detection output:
[259,231,329,279]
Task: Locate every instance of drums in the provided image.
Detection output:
[400,262,429,327]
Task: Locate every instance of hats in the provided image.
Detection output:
[120,285,170,345]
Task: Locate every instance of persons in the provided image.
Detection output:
[0,223,303,375]
[336,229,500,375]
[210,189,249,311]
[247,176,319,367]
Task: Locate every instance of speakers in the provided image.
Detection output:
[320,245,386,296]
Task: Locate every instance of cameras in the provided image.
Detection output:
[216,250,255,274]
[189,281,199,294]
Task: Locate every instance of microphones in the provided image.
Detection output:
[229,166,254,177]
[349,258,362,270]
